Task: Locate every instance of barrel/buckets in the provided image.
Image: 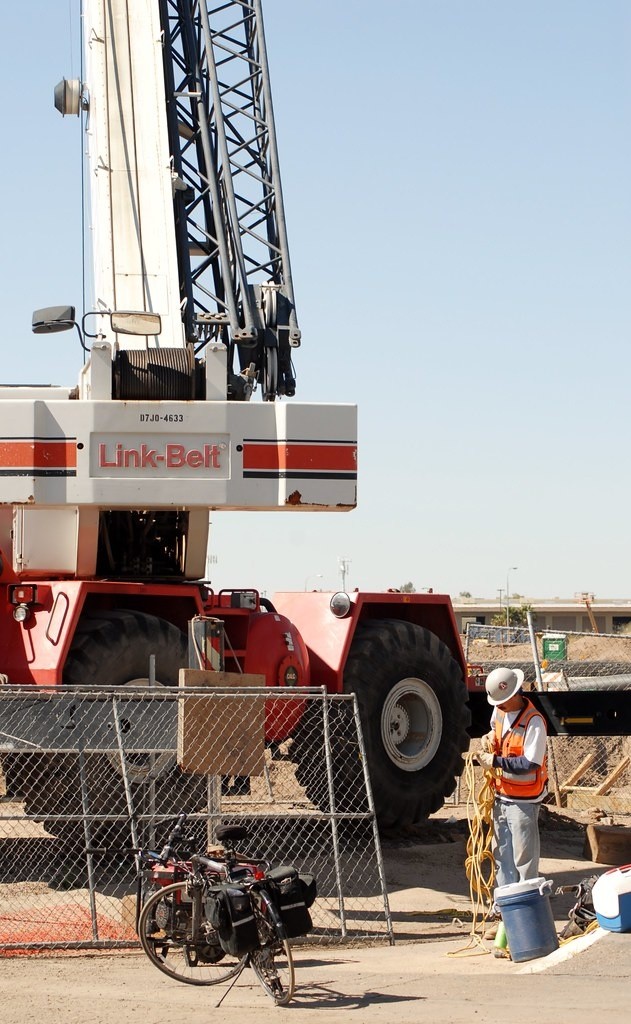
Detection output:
[494,877,559,963]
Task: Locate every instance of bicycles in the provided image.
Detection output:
[140,814,294,1010]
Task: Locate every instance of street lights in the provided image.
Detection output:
[304,573,323,592]
[505,566,516,646]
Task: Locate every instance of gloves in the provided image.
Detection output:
[481,730,495,750]
[477,754,494,769]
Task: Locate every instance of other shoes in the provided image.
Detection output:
[485,919,499,939]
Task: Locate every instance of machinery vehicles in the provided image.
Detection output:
[0,0,473,857]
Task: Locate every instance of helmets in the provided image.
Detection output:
[485,668,524,706]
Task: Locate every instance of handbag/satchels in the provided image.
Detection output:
[264,868,315,937]
[205,883,257,956]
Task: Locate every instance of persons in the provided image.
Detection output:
[474,667,550,939]
